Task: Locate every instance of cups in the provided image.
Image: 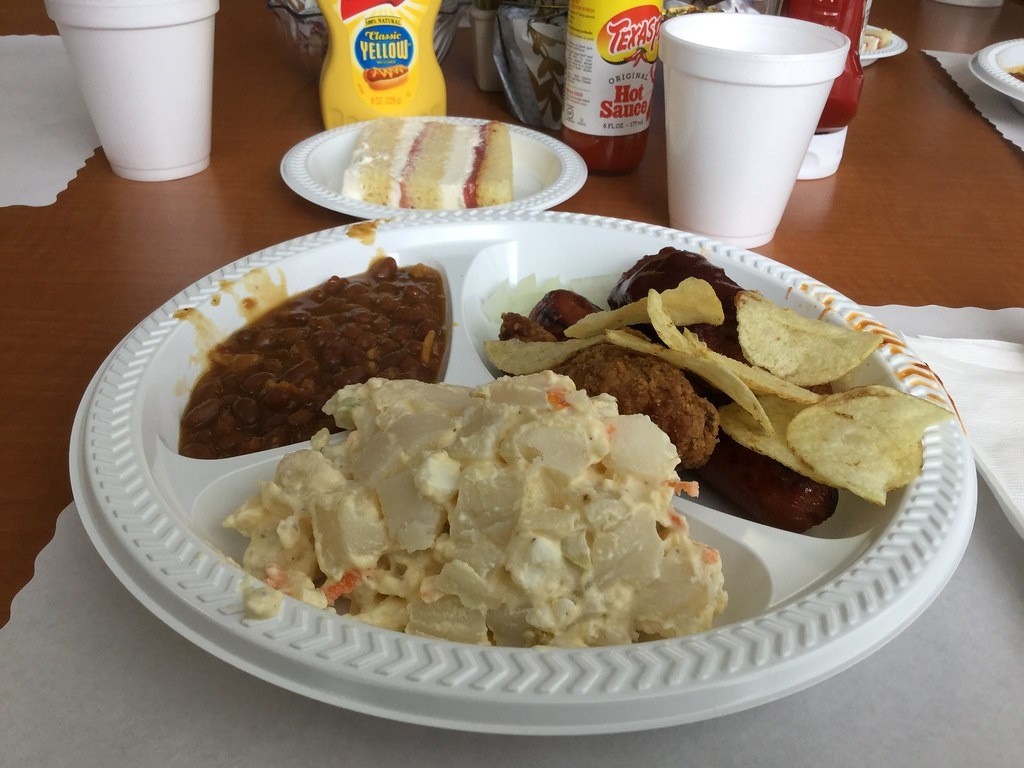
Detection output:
[657,11,850,252]
[42,0,220,182]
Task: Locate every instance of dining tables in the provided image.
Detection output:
[0,0,1024,626]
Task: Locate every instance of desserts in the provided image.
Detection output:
[343,118,513,210]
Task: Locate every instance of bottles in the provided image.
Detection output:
[779,0,873,180]
[318,0,447,131]
[561,0,663,173]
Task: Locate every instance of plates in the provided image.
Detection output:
[968,38,1024,116]
[67,210,979,735]
[855,25,909,68]
[281,116,591,221]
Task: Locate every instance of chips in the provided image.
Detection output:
[484,277,953,502]
